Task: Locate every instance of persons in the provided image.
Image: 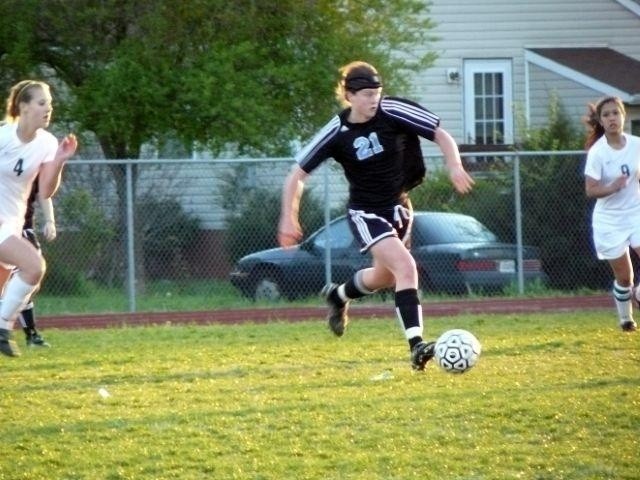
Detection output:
[0,79,78,355]
[21,182,58,350]
[581,95,640,331]
[273,59,476,373]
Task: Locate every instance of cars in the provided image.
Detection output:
[228,210,544,301]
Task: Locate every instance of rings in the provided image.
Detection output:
[52,230,55,233]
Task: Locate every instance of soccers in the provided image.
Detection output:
[434,329,480,373]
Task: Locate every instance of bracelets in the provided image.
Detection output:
[46,218,55,223]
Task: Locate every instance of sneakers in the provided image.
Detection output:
[25,332,49,350]
[322,281,349,337]
[631,286,640,309]
[410,337,435,372]
[0,332,21,357]
[621,321,638,332]
